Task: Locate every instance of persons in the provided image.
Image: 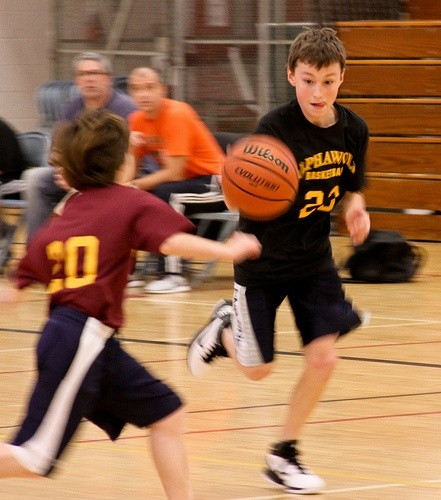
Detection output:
[0,109,261,500]
[24,52,140,235]
[114,66,226,295]
[186,27,370,494]
[0,118,40,270]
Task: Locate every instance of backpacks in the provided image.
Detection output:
[345,228,414,283]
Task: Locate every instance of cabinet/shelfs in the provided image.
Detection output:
[321,20,440,242]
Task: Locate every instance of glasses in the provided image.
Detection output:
[76,70,110,76]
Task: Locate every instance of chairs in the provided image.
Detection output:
[186,209,241,286]
[0,130,46,274]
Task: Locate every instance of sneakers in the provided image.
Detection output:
[144,272,191,294]
[187,299,232,377]
[125,272,147,287]
[261,439,325,495]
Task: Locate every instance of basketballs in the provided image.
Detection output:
[223,136,299,217]
